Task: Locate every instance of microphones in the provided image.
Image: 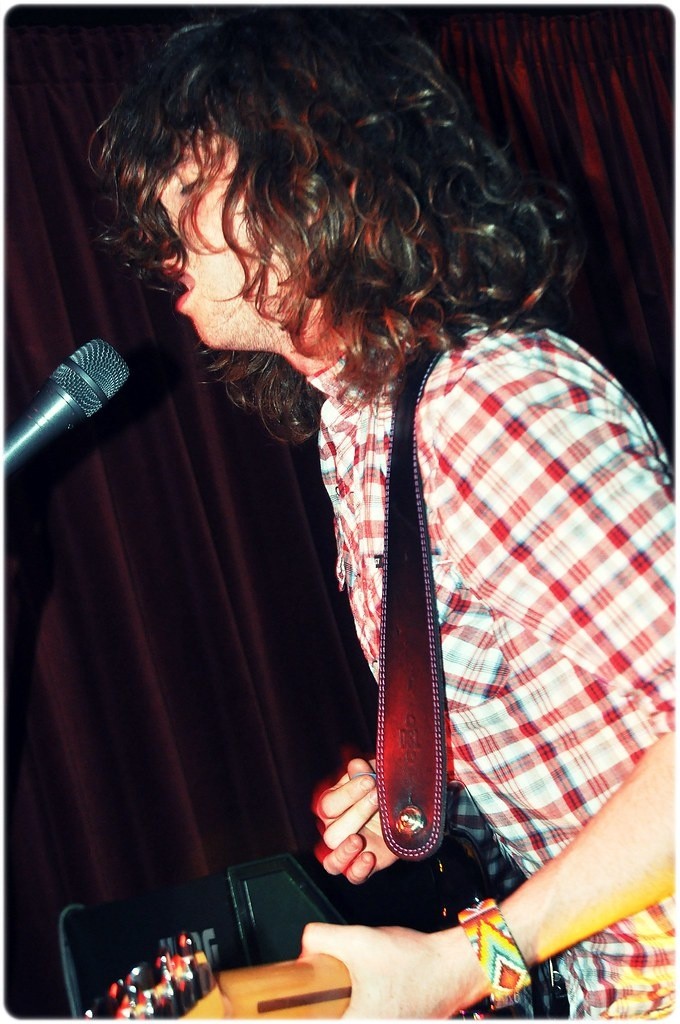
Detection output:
[5,337,130,478]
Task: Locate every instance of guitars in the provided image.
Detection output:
[84,868,516,1018]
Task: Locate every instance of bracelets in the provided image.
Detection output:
[458,898,532,1003]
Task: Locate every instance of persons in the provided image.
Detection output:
[87,5,676,1020]
